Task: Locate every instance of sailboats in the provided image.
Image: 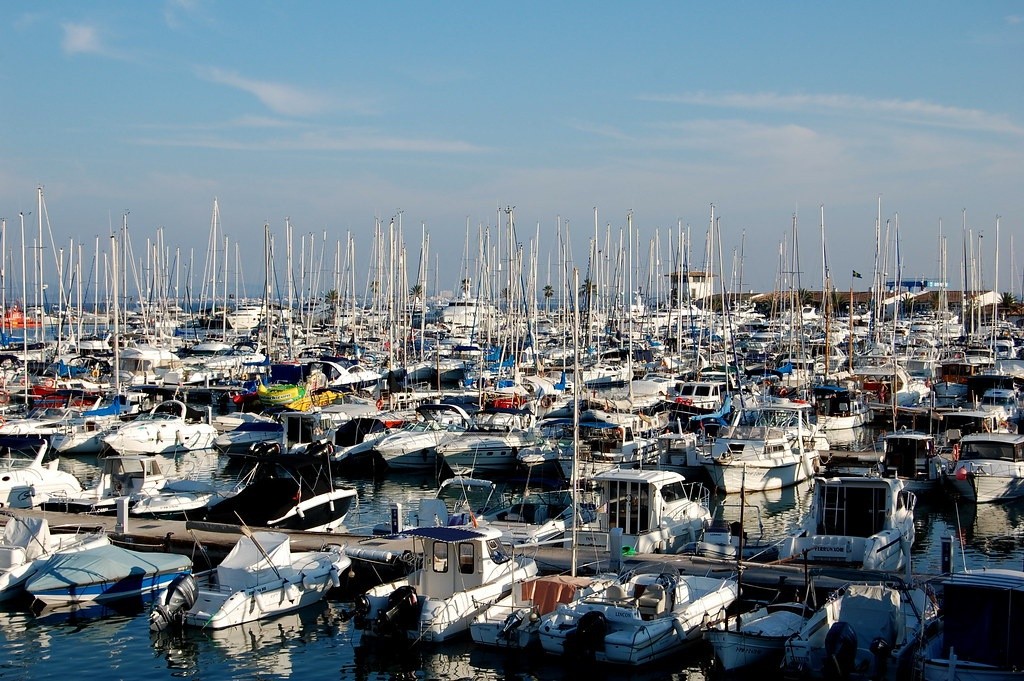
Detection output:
[0,186,1024,680]
